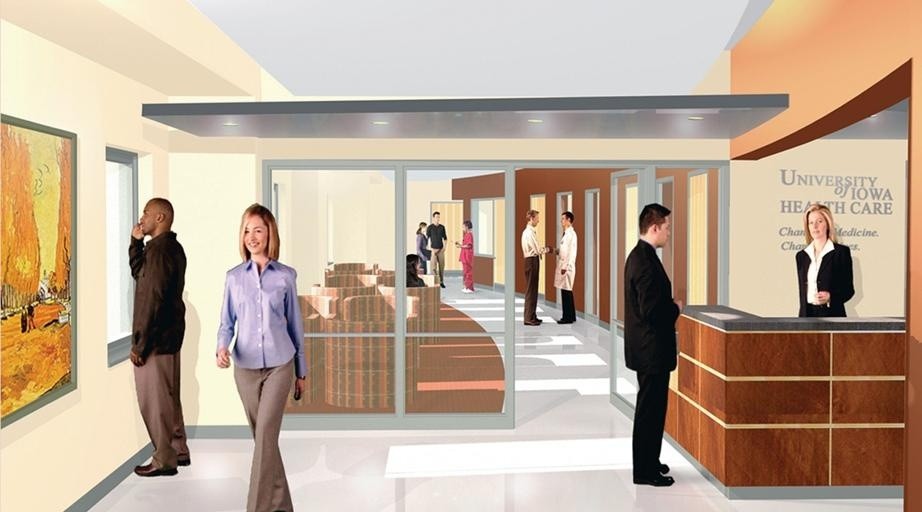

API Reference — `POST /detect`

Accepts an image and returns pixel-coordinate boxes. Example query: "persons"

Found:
[128,198,190,476]
[426,211,447,288]
[521,210,549,326]
[623,203,684,486]
[216,203,308,512]
[795,204,855,317]
[21,303,34,333]
[550,212,578,324]
[406,254,428,286]
[455,220,476,292]
[415,222,428,275]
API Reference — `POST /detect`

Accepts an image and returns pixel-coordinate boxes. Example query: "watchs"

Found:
[297,376,306,380]
